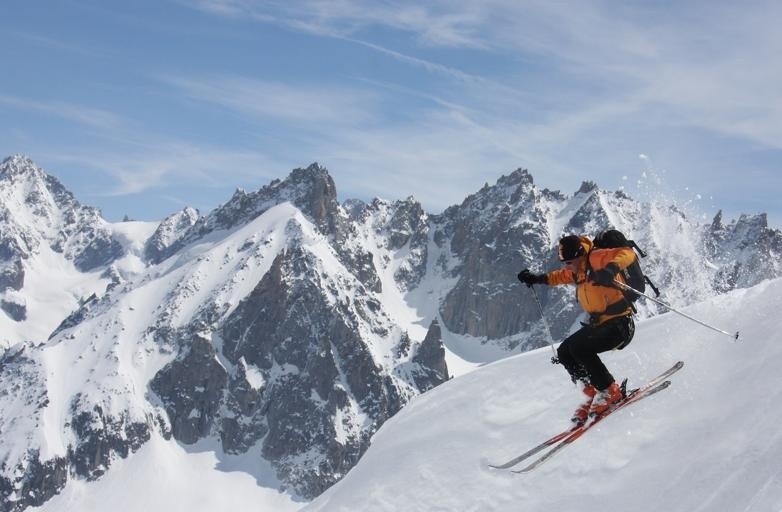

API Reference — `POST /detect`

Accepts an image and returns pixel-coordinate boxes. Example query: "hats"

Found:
[558,235,587,262]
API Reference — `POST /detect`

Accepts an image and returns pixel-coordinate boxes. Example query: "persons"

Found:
[518,229,640,424]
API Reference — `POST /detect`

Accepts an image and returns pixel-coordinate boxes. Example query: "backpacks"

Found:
[571,229,645,302]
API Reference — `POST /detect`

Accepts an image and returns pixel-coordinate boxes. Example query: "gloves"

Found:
[517,268,549,287]
[589,262,620,287]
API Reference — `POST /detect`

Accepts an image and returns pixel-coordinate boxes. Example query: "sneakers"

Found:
[571,384,598,423]
[588,382,624,418]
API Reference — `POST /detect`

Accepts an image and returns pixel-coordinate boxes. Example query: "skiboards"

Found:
[487,361,684,473]
